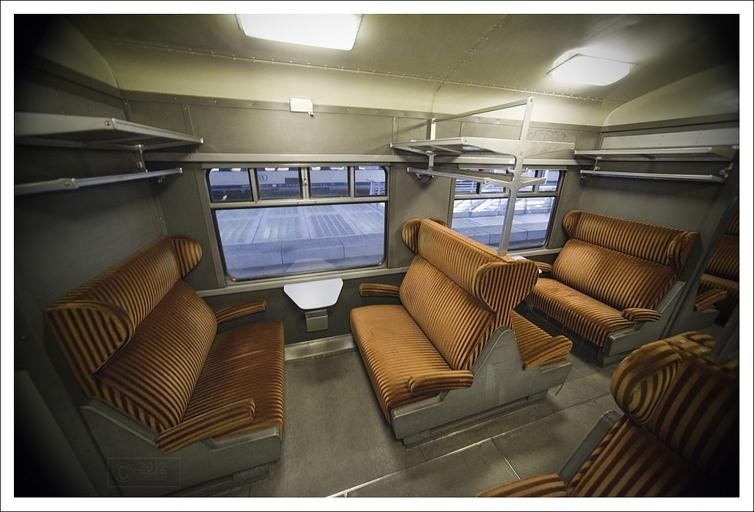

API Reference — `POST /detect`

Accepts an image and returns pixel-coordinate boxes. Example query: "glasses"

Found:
[229,13,363,52]
[546,51,638,87]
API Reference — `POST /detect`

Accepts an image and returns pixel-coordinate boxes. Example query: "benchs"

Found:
[348,207,739,447]
[39,233,286,497]
[475,331,739,498]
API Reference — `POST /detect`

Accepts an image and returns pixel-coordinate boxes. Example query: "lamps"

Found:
[546,51,638,87]
[229,13,363,52]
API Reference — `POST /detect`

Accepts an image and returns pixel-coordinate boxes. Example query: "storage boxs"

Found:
[39,233,286,497]
